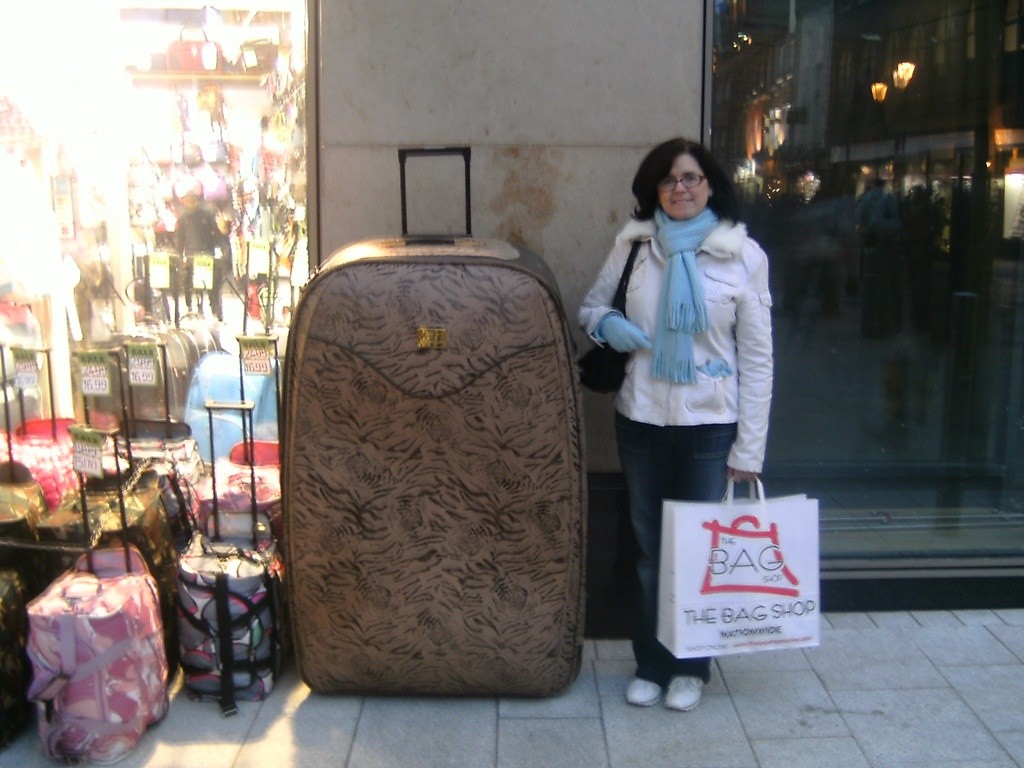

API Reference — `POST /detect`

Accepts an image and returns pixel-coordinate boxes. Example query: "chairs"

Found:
[243,222,301,334]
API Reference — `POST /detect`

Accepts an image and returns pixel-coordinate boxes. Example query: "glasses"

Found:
[657,174,707,190]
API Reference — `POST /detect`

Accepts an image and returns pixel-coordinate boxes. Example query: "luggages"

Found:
[0,309,281,768]
[280,145,589,699]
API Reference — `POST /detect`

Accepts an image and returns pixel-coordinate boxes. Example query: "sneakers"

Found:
[626,678,662,707]
[664,676,705,712]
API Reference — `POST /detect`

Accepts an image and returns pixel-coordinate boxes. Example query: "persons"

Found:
[170,192,231,327]
[725,164,945,341]
[576,136,774,710]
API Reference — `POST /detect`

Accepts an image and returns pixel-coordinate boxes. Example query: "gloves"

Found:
[699,358,732,380]
[599,314,652,352]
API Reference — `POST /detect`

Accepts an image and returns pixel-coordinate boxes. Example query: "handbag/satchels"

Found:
[115,17,279,236]
[656,475,820,659]
[576,240,643,394]
